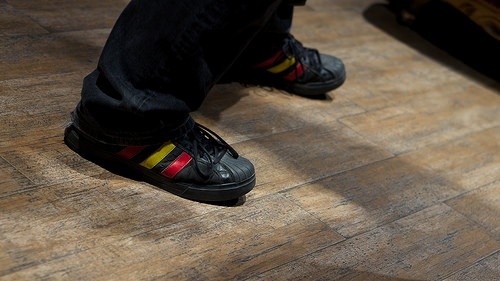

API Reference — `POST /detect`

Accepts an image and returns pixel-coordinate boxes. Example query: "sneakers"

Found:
[65,98,256,199]
[245,33,346,92]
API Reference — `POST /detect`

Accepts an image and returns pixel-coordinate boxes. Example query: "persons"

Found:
[64,0,347,204]
